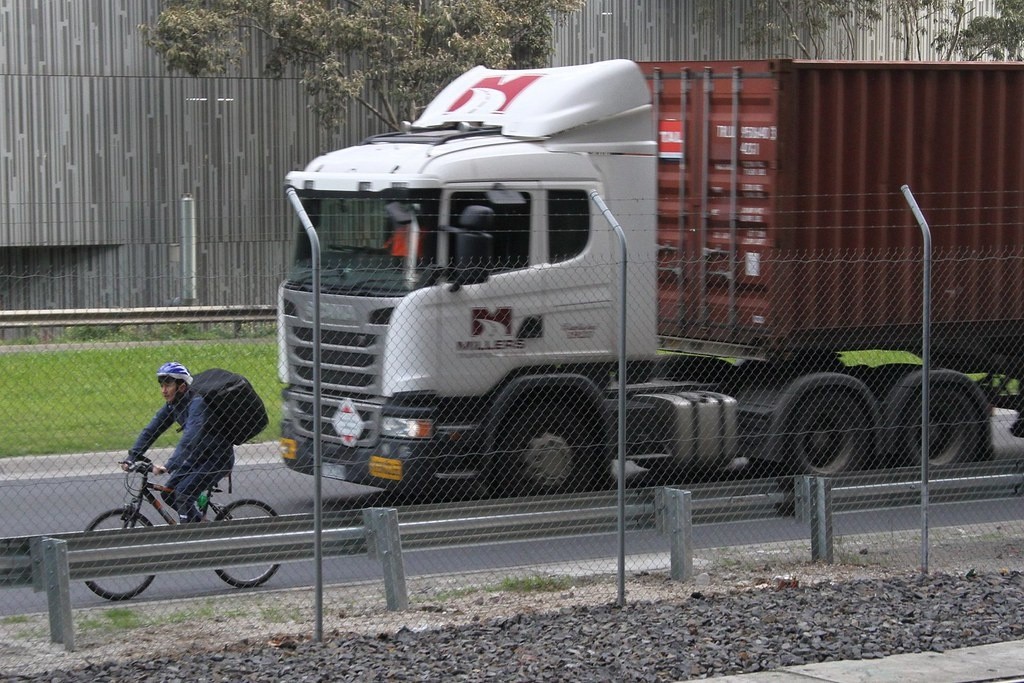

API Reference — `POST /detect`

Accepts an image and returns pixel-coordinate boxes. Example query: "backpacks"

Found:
[181,368,270,446]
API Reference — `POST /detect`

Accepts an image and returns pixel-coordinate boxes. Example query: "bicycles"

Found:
[79,455,288,600]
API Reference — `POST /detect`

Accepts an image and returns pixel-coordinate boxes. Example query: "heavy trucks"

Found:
[270,57,1024,503]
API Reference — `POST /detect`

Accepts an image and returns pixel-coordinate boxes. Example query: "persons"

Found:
[122,362,234,523]
[381,202,427,256]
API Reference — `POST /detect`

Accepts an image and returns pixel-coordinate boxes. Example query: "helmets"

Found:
[157,362,193,385]
